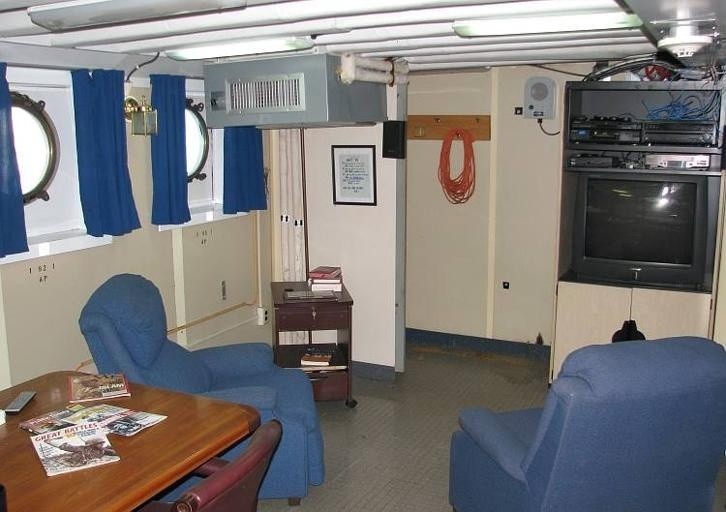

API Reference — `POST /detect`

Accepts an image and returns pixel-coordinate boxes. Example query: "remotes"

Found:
[5,392,36,414]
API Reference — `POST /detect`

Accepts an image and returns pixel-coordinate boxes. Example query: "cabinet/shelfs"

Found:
[271,282,356,407]
[548,282,715,385]
[562,81,726,175]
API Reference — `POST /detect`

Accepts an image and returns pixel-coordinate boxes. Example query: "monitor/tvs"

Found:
[572,173,708,284]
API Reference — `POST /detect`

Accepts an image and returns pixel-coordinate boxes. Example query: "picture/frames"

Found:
[331,145,377,206]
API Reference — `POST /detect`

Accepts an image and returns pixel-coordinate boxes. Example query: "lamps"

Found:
[165,38,311,61]
[452,15,643,38]
[27,0,246,31]
[124,95,161,139]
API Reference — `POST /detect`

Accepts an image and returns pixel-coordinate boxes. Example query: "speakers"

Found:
[383,121,405,158]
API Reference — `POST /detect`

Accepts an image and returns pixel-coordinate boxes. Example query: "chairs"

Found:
[450,337,726,512]
[78,274,324,505]
[133,419,284,512]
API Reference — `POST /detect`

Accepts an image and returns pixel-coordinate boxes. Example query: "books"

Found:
[300,350,333,365]
[18,372,168,477]
[308,266,343,292]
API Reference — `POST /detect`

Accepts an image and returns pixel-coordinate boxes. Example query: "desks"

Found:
[0,372,259,512]
